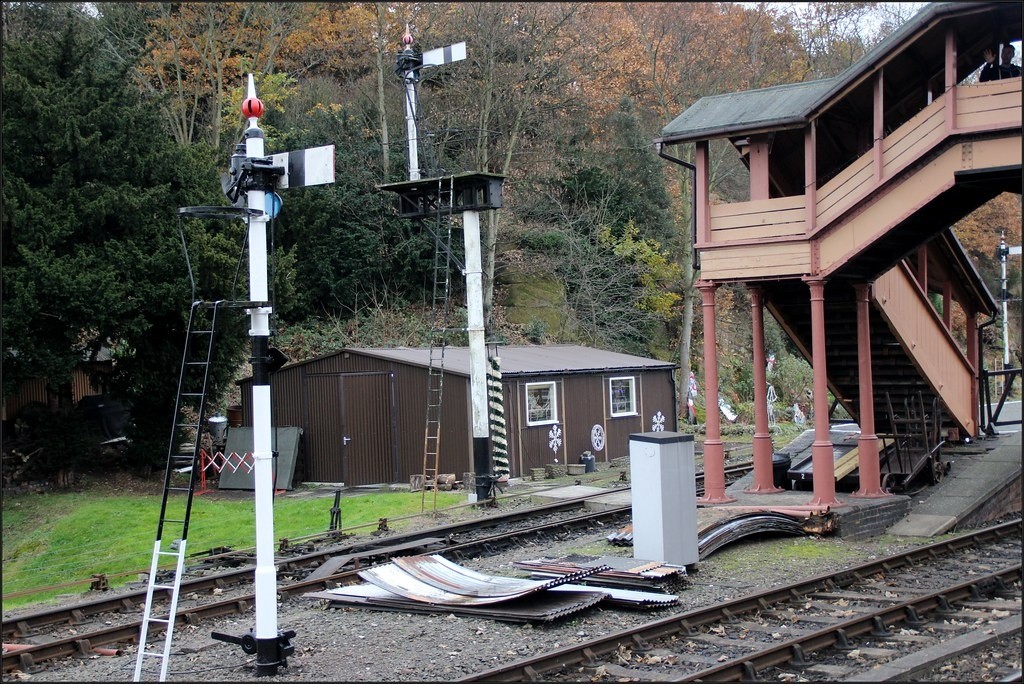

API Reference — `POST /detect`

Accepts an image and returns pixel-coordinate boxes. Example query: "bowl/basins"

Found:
[567,464,586,476]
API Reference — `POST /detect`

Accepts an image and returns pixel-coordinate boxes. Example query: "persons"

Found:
[979,44,1022,82]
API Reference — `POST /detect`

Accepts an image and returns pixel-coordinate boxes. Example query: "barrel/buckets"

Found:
[580,454,595,473]
